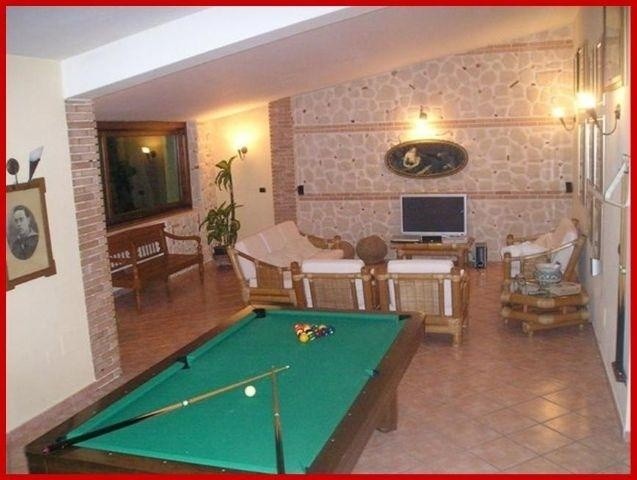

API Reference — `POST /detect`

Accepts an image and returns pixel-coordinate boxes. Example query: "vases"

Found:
[533,261,563,284]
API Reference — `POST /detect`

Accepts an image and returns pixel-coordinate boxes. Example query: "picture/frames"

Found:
[6,175,58,293]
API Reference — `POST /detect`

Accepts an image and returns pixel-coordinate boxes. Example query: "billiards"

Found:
[293,323,335,343]
[243,386,256,396]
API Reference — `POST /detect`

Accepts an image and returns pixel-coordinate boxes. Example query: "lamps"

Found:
[552,106,579,133]
[415,102,431,123]
[141,145,157,159]
[7,144,46,184]
[236,145,248,160]
[576,92,623,137]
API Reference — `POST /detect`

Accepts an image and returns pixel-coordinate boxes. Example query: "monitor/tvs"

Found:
[401,194,467,245]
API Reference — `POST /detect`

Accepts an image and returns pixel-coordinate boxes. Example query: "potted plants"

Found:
[198,149,247,269]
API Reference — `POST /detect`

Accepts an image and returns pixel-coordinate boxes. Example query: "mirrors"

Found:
[95,119,195,227]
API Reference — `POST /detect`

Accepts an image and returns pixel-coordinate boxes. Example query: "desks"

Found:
[388,236,477,259]
[24,300,428,478]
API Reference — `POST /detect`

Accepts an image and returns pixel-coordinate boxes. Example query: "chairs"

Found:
[290,259,380,314]
[374,257,471,349]
[498,216,591,291]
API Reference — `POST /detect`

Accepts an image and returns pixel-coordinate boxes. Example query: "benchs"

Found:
[105,222,205,312]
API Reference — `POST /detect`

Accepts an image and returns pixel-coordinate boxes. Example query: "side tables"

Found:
[500,281,593,338]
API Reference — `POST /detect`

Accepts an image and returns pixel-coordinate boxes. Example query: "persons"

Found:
[395,146,424,175]
[9,206,38,259]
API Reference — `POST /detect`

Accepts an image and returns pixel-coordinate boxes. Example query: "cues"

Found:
[42,366,288,474]
[616,173,630,371]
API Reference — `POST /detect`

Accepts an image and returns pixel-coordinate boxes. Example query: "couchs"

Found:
[225,218,356,308]
